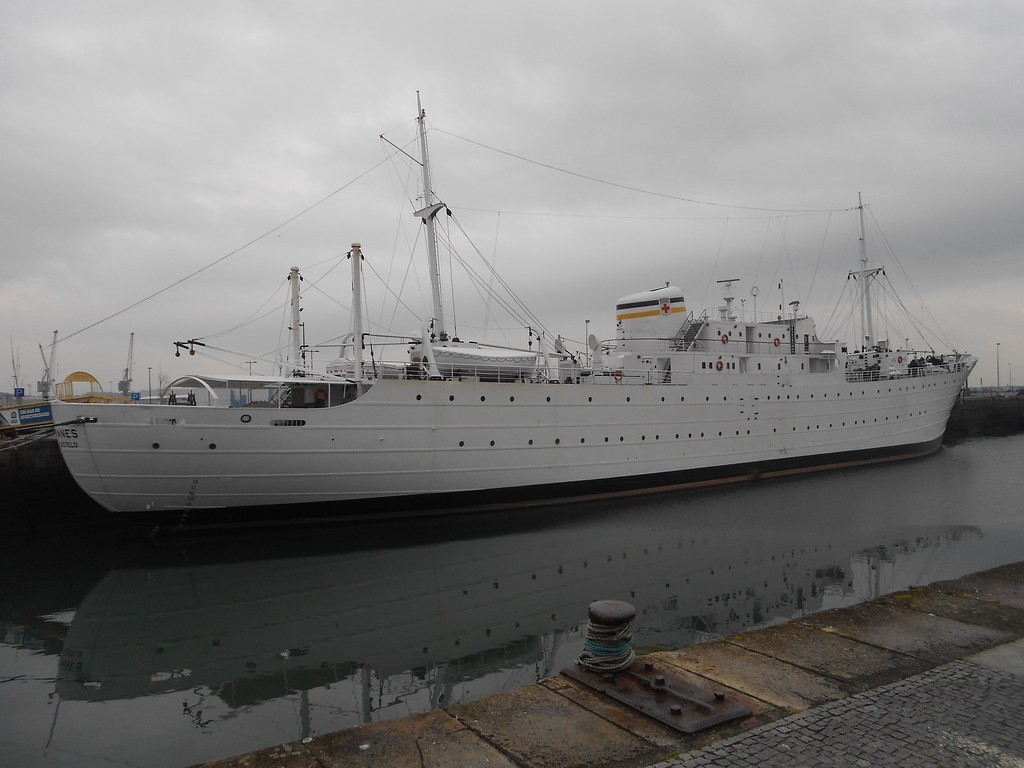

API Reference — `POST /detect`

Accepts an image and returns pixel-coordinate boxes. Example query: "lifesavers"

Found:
[315,389,328,402]
[774,338,780,347]
[898,356,902,363]
[722,334,728,344]
[716,361,723,371]
[614,370,622,381]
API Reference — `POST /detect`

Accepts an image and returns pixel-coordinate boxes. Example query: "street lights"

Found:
[996,342,1001,390]
[306,349,319,378]
[245,362,257,402]
[1008,363,1011,390]
[585,319,590,369]
[148,368,153,405]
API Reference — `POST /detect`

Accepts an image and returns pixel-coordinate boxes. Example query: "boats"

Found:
[35,88,980,549]
[44,446,988,755]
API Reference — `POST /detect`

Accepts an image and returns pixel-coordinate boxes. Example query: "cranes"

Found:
[118,332,134,396]
[36,330,58,401]
